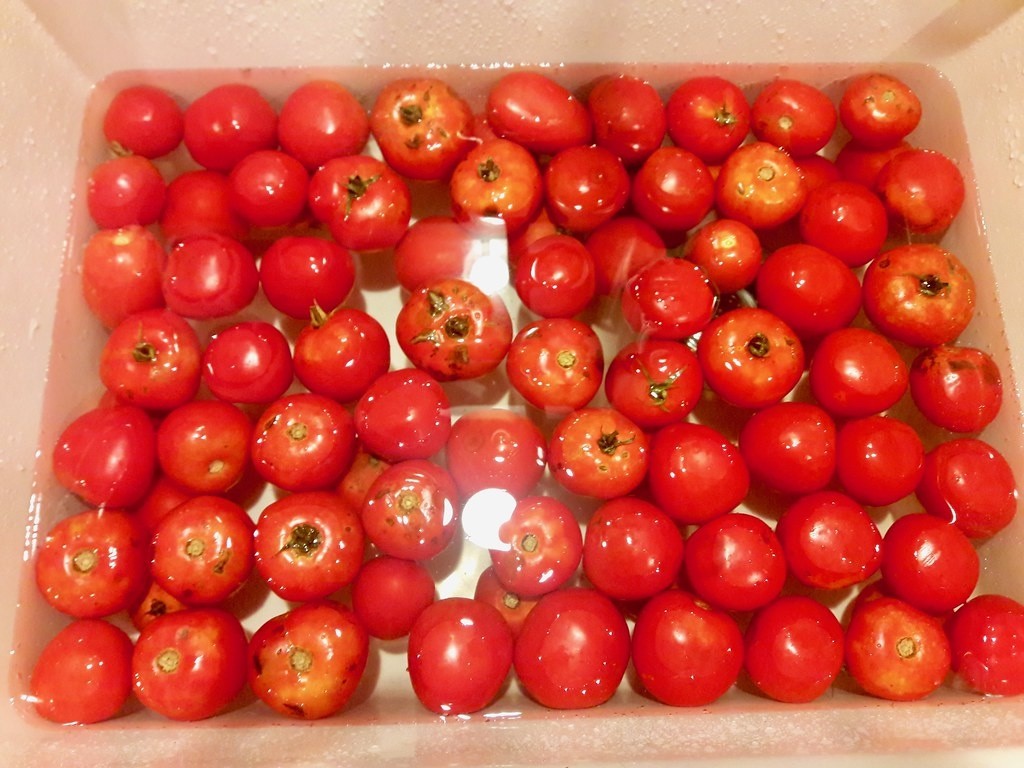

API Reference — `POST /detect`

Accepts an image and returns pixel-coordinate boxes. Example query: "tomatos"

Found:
[28,70,1024,723]
